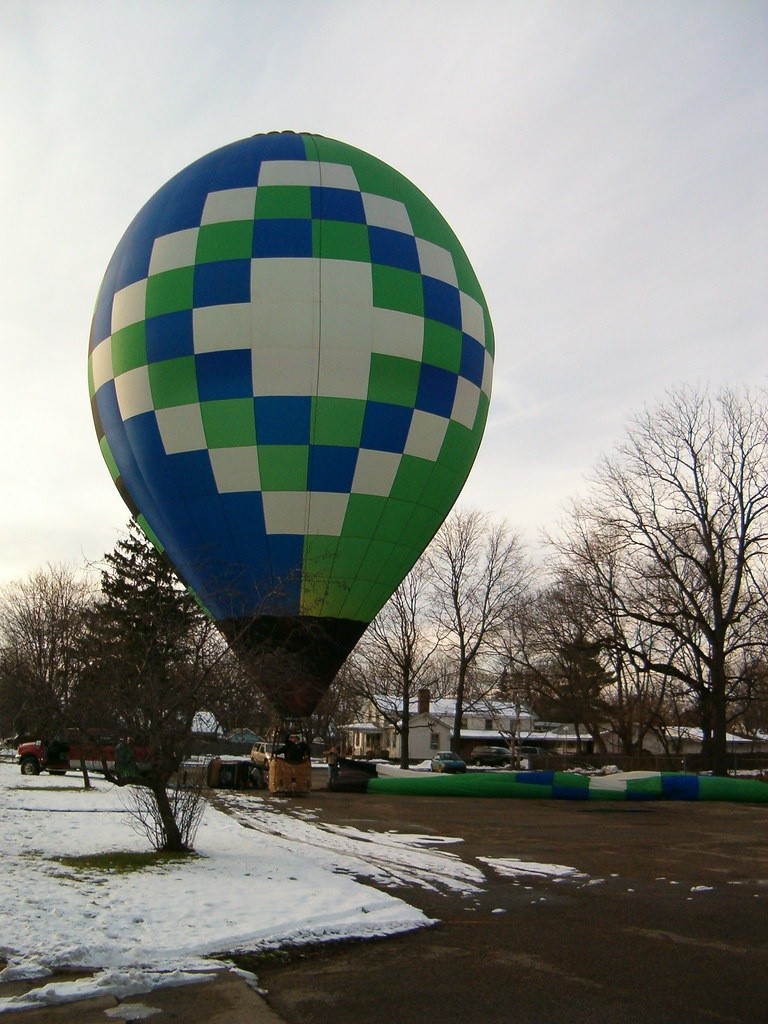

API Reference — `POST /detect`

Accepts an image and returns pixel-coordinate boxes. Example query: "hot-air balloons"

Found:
[87,131,494,793]
[310,753,768,807]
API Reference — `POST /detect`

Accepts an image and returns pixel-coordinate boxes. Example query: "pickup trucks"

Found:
[14,726,154,782]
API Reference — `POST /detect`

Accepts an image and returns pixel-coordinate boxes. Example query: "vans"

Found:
[251,741,286,768]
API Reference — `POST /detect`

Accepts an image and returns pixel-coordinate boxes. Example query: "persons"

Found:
[114,736,135,769]
[273,738,304,764]
[322,747,339,784]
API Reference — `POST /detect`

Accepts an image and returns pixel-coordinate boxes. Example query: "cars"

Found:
[429,751,468,773]
[469,745,514,767]
[514,747,553,757]
